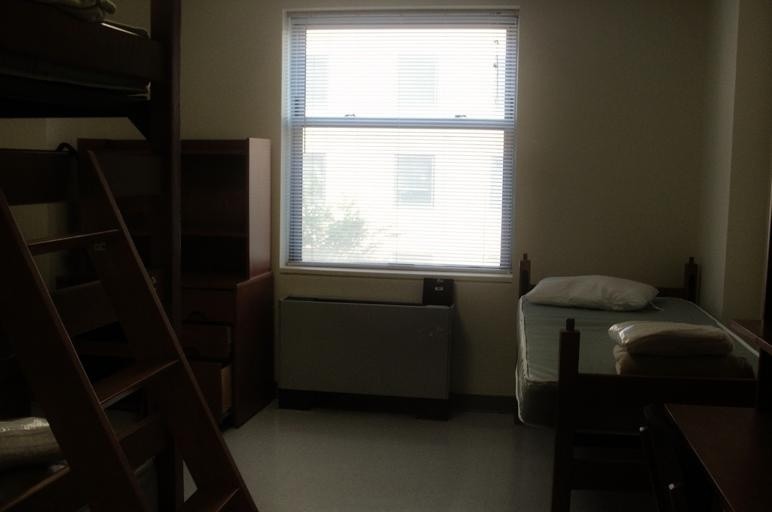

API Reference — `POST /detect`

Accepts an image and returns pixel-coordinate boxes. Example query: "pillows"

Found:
[609,314,735,356]
[527,270,661,313]
[610,345,756,381]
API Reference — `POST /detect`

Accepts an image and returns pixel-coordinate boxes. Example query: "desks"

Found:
[662,394,771,511]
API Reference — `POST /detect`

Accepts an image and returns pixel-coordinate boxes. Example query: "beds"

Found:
[0,0,264,509]
[507,253,770,512]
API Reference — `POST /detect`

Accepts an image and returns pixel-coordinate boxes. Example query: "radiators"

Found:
[273,296,458,424]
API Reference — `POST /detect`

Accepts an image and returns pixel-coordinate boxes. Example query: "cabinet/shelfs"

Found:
[55,270,277,429]
[72,134,273,280]
